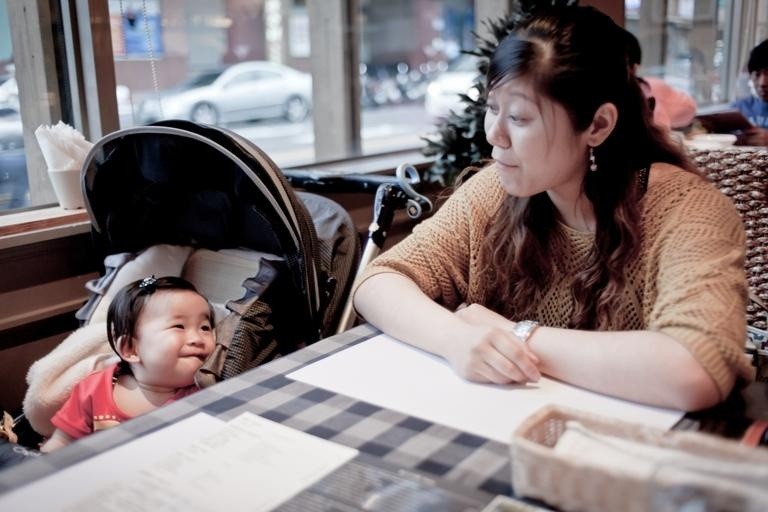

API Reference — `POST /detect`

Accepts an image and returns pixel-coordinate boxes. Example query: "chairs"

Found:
[689,149,768,380]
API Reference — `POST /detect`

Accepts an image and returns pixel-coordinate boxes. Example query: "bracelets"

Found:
[510,316,540,343]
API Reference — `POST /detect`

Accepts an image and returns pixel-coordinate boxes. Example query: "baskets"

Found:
[513,403,767,512]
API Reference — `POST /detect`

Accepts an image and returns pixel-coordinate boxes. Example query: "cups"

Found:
[48,170,83,211]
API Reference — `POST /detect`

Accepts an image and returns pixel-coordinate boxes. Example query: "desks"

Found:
[0,319,767,512]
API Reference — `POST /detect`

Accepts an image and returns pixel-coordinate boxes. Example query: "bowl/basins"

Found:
[691,134,737,146]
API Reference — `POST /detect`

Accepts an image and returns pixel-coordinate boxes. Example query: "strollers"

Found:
[0,121,435,470]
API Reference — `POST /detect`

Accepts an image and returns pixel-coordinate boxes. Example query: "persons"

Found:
[40,275,220,455]
[347,5,752,416]
[621,28,697,136]
[731,36,768,147]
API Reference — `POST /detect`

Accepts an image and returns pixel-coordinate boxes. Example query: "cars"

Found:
[139,61,312,125]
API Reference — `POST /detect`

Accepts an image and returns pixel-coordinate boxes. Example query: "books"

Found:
[693,108,757,142]
